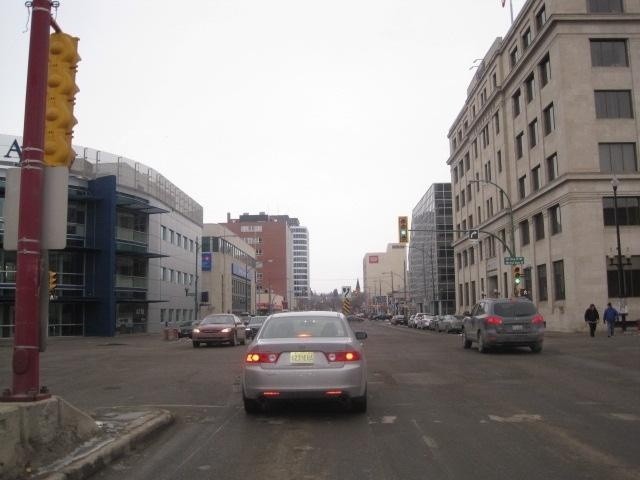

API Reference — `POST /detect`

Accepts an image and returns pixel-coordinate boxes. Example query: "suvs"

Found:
[461,298,545,354]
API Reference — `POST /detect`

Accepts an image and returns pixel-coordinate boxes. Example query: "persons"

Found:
[584,304,599,337]
[603,302,619,337]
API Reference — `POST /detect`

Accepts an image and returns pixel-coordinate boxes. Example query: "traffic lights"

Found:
[49,270,57,292]
[398,216,409,243]
[520,289,528,296]
[514,265,520,285]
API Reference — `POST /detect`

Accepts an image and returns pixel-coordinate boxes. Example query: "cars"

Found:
[232,311,269,341]
[347,312,465,334]
[178,320,201,338]
[115,315,134,334]
[192,313,246,348]
[242,310,368,415]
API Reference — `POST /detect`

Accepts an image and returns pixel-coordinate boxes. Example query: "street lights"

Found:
[504,257,525,265]
[195,234,241,320]
[409,245,436,315]
[469,179,519,297]
[607,173,632,332]
[246,259,274,312]
[382,272,406,316]
